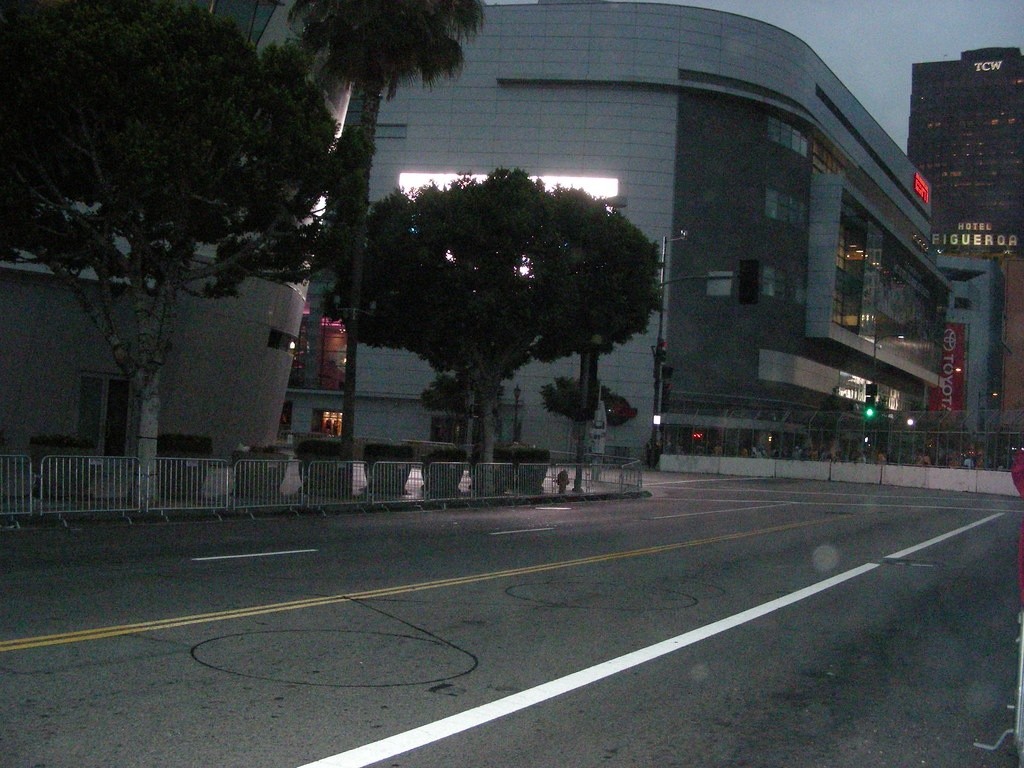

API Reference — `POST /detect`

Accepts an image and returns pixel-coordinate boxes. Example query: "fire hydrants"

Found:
[557,469,570,494]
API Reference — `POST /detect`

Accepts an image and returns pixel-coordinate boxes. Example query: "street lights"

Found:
[650,232,689,470]
[512,382,521,447]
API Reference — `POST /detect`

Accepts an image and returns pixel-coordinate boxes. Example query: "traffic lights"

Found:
[661,366,673,379]
[865,397,873,418]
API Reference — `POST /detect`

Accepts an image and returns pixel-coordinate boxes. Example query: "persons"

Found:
[557,468,570,494]
[676,443,983,470]
